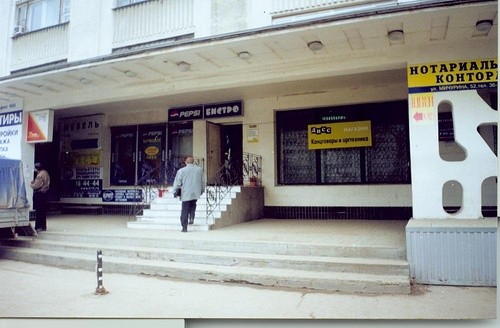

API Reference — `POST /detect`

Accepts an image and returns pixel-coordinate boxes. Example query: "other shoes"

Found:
[181,227,188,232]
[189,221,193,224]
[34,228,46,232]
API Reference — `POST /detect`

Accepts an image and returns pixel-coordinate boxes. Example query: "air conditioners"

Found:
[13,26,25,35]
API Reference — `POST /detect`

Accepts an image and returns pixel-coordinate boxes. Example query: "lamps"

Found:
[177,62,190,72]
[388,30,403,40]
[308,41,322,50]
[476,20,493,32]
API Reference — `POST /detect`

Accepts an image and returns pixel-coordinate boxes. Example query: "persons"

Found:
[29,162,50,232]
[172,157,205,233]
